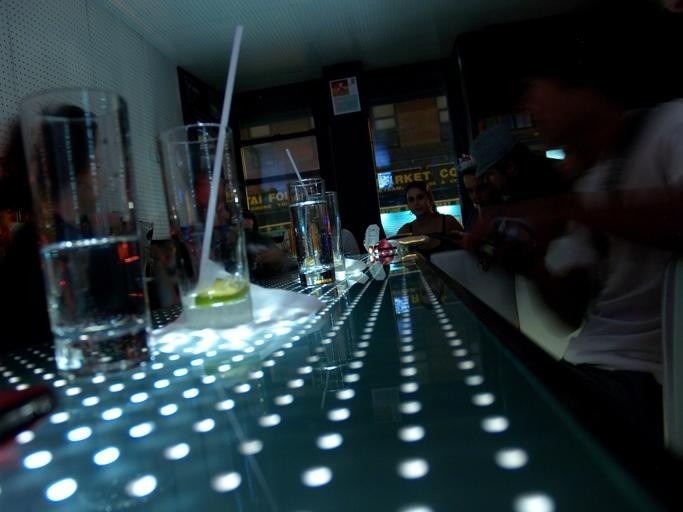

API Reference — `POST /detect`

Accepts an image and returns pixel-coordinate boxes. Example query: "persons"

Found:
[0,104,129,350]
[456,0,683,413]
[395,179,462,237]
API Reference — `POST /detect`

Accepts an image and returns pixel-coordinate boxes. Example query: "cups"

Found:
[23,89,154,379]
[160,122,252,329]
[287,178,346,287]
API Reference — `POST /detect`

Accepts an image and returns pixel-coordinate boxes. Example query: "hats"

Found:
[471,131,519,177]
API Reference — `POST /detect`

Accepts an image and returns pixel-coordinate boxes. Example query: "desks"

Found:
[0,252,658,511]
